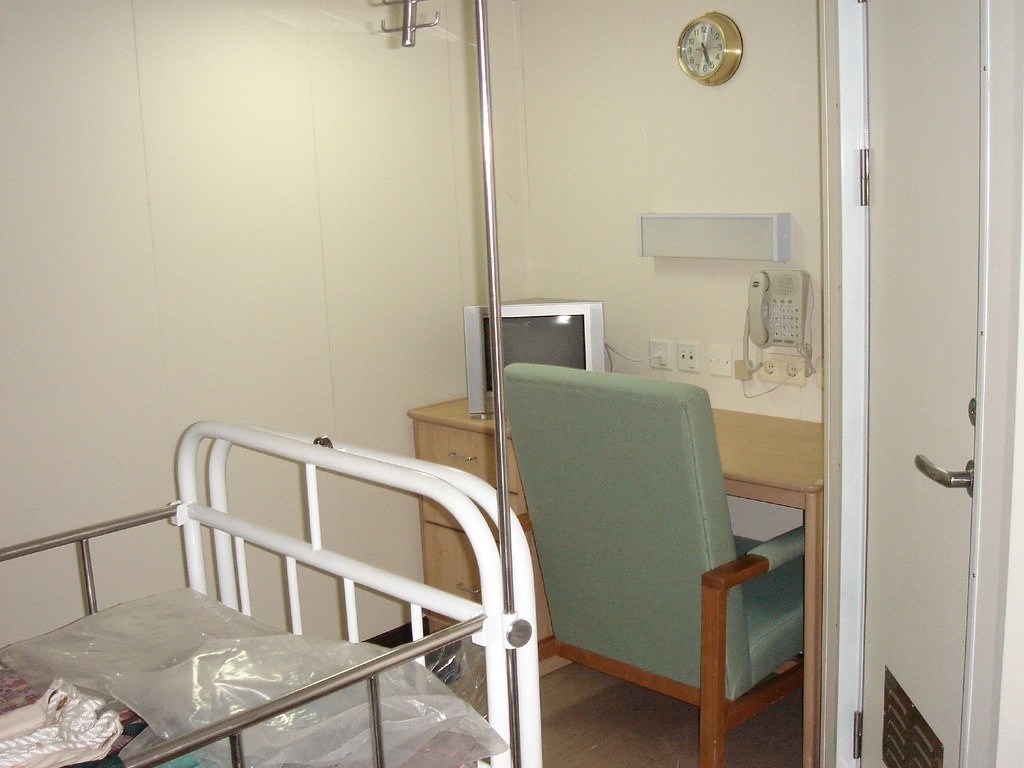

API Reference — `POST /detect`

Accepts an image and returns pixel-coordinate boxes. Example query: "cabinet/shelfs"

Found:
[406,397,823,768]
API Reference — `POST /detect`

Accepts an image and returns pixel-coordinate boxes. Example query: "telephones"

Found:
[744,266,813,350]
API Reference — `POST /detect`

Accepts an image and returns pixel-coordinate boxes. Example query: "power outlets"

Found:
[706,344,734,377]
[648,340,673,370]
[676,341,701,373]
[756,352,807,386]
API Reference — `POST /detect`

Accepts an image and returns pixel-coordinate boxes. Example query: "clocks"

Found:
[676,9,743,87]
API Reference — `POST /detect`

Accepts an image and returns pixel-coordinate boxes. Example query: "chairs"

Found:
[502,362,805,768]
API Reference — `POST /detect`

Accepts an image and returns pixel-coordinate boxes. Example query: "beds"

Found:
[0,418,544,768]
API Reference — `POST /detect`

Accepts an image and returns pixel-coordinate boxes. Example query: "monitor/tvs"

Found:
[463,297,605,420]
